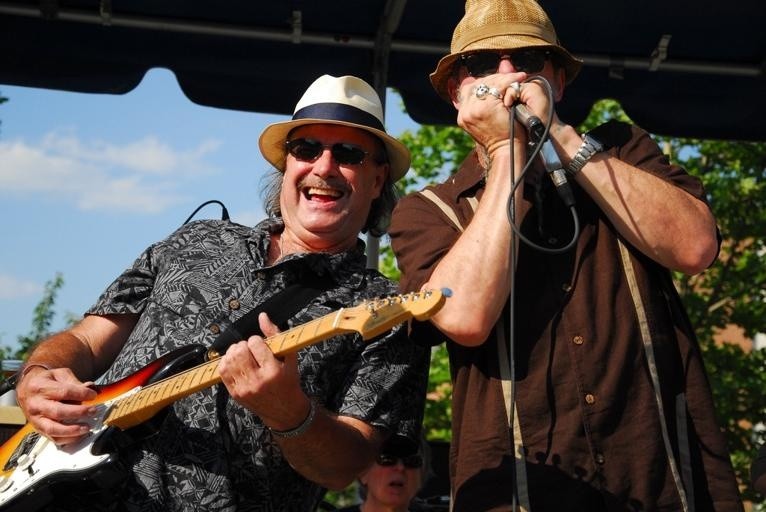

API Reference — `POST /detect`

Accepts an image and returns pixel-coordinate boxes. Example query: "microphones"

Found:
[508,101,546,143]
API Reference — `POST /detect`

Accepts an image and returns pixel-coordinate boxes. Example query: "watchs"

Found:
[566,133,603,176]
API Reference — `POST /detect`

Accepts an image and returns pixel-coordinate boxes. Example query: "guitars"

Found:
[0,288,452,512]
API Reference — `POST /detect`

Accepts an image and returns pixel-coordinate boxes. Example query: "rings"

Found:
[511,82,520,100]
[475,84,488,99]
[488,88,500,99]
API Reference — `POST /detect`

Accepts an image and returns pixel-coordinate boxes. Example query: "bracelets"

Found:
[269,399,315,440]
[22,363,51,378]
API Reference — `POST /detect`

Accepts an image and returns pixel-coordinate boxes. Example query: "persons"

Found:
[339,430,450,512]
[0,74,429,512]
[388,0,745,512]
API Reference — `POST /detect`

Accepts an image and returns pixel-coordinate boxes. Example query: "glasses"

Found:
[286,137,370,164]
[460,47,553,78]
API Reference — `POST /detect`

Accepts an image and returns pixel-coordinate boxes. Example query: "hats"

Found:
[427,0,587,105]
[258,73,412,185]
